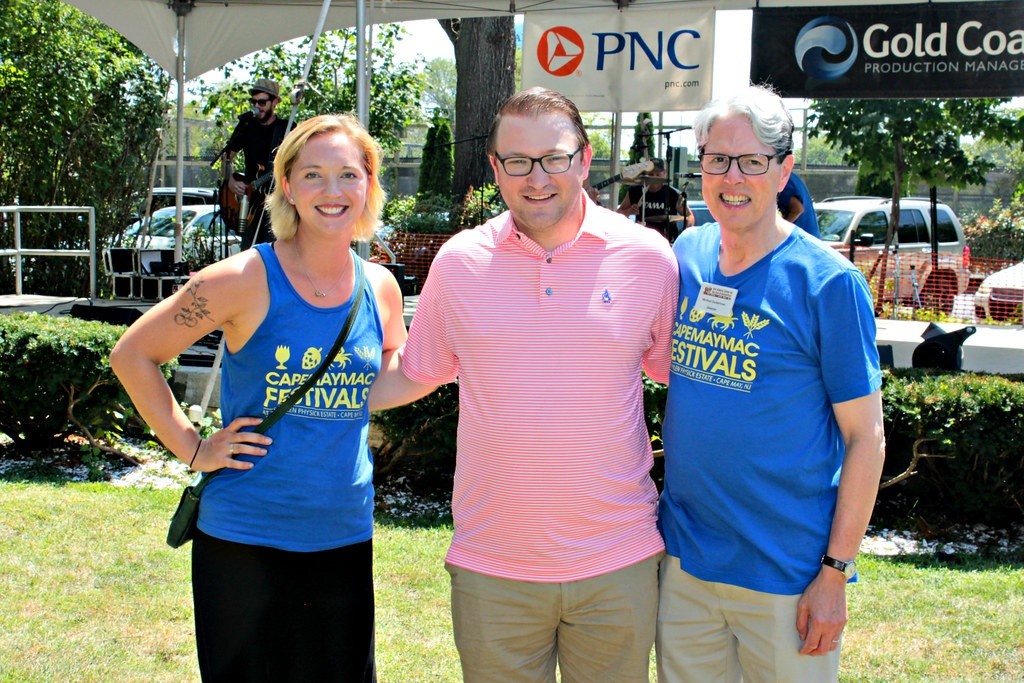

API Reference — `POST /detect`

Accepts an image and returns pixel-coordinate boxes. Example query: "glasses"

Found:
[698,149,789,175]
[249,98,270,106]
[494,146,583,177]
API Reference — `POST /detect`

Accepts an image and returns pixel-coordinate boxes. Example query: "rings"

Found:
[229,444,235,455]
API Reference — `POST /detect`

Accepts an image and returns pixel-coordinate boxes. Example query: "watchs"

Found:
[821,552,858,581]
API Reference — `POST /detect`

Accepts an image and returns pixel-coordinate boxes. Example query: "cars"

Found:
[113,206,241,260]
[126,187,218,209]
[973,257,1023,327]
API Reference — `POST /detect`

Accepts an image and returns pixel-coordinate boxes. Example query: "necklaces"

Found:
[293,233,353,297]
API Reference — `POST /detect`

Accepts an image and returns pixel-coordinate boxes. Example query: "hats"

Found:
[650,158,664,172]
[248,79,282,103]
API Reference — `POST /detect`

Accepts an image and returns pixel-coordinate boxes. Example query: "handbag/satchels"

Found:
[166,472,205,549]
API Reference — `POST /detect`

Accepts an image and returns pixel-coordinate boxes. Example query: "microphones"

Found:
[238,110,260,121]
[676,173,702,178]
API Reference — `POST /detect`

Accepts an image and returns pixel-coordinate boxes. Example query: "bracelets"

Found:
[189,439,203,468]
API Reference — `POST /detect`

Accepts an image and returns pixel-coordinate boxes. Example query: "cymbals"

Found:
[637,176,672,182]
[645,215,689,222]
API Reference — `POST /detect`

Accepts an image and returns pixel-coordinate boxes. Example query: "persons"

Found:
[107,110,412,683]
[655,86,885,683]
[617,154,694,252]
[776,172,820,240]
[221,76,301,250]
[364,86,683,683]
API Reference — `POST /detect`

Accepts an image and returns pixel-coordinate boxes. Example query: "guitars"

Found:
[219,168,274,229]
[584,156,654,193]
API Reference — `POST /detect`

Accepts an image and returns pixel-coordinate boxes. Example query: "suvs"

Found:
[814,194,970,317]
